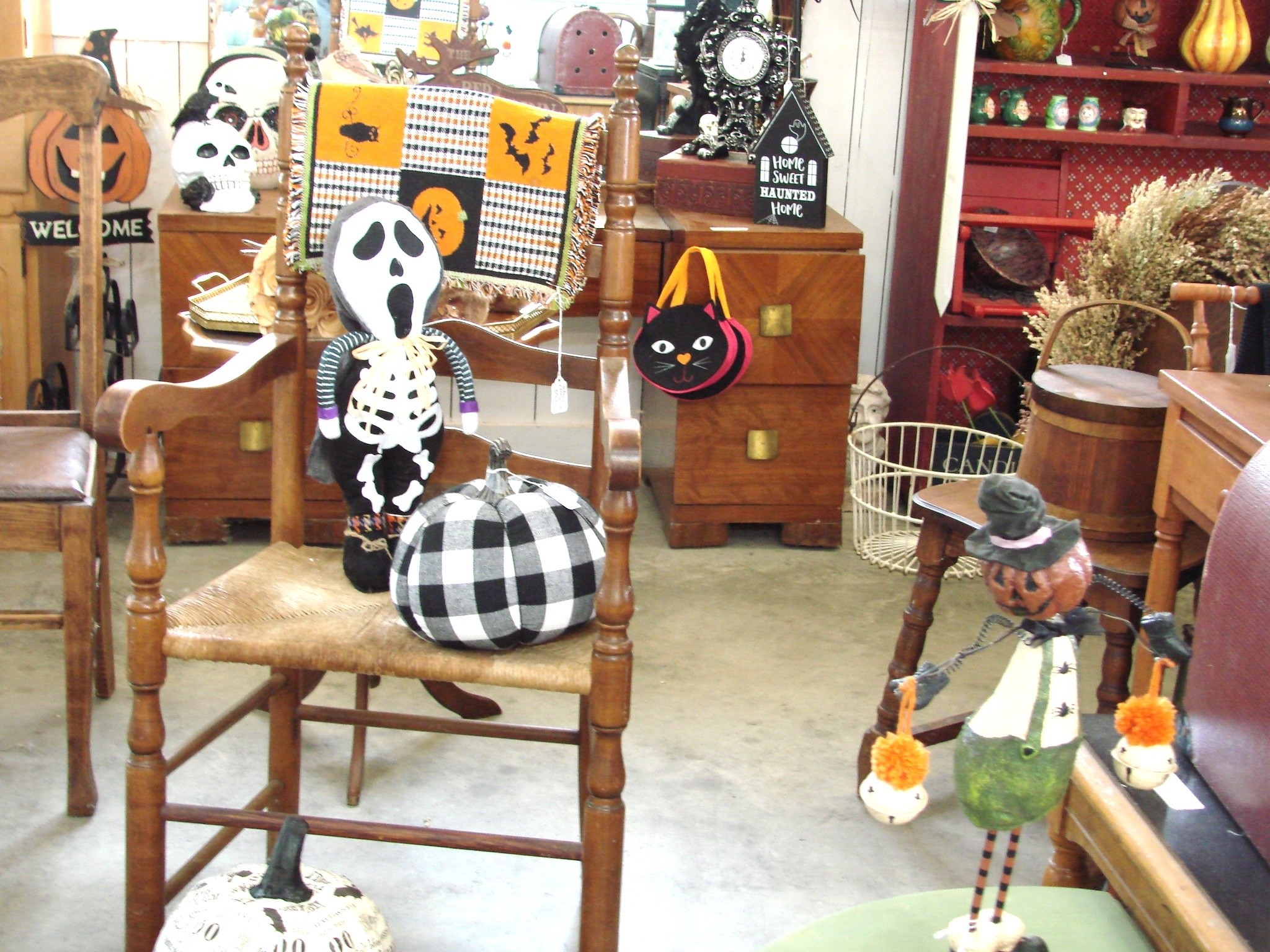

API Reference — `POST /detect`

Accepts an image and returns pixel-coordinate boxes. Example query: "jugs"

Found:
[1217,94,1266,139]
[985,0,1082,62]
[999,84,1032,127]
[969,84,994,125]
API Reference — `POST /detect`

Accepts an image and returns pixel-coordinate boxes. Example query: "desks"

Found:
[1044,366,1269,951]
[158,184,865,549]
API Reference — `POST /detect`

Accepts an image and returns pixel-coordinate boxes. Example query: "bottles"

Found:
[1045,94,1069,129]
[1076,96,1101,132]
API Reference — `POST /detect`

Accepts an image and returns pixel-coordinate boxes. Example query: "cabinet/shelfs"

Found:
[885,1,1269,516]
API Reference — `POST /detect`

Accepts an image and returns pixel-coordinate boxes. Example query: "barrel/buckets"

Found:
[1016,300,1193,544]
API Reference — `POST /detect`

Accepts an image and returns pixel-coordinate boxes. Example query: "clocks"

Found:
[684,1,799,164]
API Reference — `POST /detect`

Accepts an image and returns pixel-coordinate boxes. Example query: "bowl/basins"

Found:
[966,206,1050,292]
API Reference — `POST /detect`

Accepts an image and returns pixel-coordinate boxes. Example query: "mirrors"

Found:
[335,0,805,198]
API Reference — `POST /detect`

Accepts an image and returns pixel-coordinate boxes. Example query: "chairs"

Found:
[855,184,1265,796]
[92,23,639,952]
[1,56,119,823]
